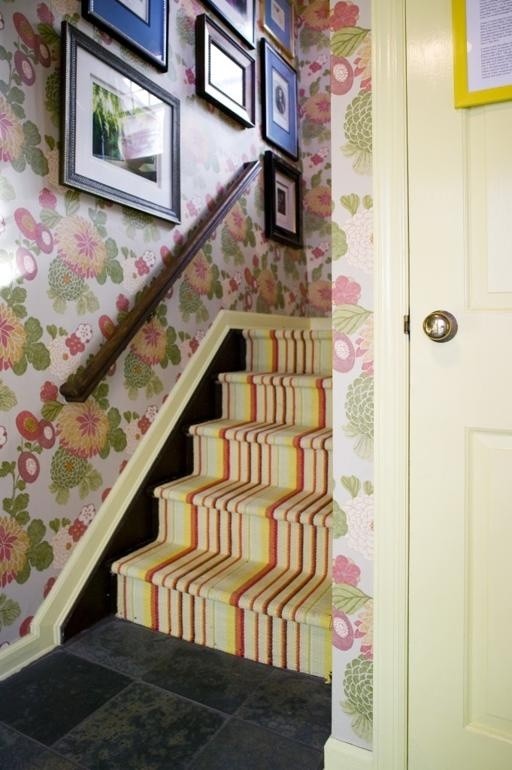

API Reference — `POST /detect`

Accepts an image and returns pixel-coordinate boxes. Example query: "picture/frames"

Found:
[200,0,257,50]
[261,0,295,59]
[260,37,299,162]
[264,150,303,249]
[196,13,256,128]
[82,0,169,73]
[451,0,512,109]
[59,19,182,225]
